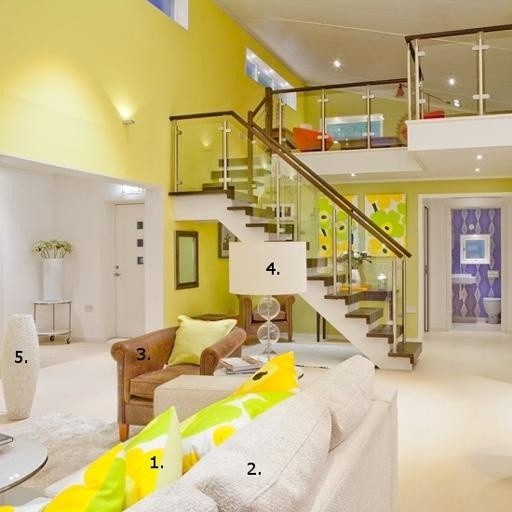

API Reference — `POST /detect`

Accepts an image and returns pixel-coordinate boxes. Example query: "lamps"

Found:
[394,82,451,114]
[123,119,136,125]
[228,239,309,374]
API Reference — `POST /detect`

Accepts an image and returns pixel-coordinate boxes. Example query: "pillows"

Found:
[180,392,297,478]
[167,313,237,368]
[125,407,187,507]
[226,352,300,394]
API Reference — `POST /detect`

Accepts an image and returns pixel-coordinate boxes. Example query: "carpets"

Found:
[0,407,145,509]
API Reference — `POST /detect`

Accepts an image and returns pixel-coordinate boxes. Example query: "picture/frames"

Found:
[460,234,490,266]
[174,229,199,291]
[218,222,237,258]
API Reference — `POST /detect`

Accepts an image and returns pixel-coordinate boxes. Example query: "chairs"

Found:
[237,293,294,340]
[112,312,248,443]
[402,109,446,141]
[292,127,333,151]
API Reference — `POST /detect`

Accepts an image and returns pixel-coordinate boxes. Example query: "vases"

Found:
[42,263,64,303]
[351,269,360,287]
[0,311,40,422]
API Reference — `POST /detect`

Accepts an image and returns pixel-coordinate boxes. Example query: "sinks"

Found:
[451,276,480,285]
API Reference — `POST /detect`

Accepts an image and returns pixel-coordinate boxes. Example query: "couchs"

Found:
[0,353,410,512]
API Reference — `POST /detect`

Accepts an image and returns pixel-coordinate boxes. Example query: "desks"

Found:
[336,136,407,149]
[316,289,399,342]
[264,128,295,152]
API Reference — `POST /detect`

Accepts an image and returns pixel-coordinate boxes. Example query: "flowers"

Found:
[33,239,73,264]
[337,245,372,269]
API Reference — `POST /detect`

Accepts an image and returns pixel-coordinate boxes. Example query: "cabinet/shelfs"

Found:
[33,300,72,345]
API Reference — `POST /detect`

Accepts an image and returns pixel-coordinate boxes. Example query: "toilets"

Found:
[483,297,501,324]
